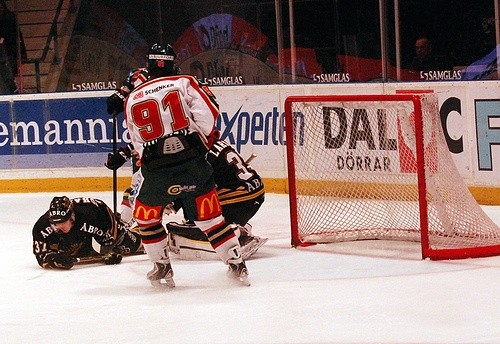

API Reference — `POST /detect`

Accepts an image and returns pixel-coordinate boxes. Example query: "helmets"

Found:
[49,196,74,224]
[148,41,176,70]
[123,67,151,93]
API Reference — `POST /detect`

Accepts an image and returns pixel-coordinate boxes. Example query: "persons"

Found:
[124,43,251,288]
[32,196,144,270]
[409,38,442,81]
[105,67,196,225]
[167,124,267,260]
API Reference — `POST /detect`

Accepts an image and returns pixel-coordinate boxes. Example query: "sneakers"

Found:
[238,227,269,259]
[147,246,175,288]
[227,245,250,286]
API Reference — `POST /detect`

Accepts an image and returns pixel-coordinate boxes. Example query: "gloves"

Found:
[105,254,122,264]
[43,252,73,270]
[107,88,128,117]
[105,147,130,170]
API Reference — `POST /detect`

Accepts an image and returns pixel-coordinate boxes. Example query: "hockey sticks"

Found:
[101,216,137,256]
[63,251,114,264]
[245,153,258,166]
[111,115,119,214]
